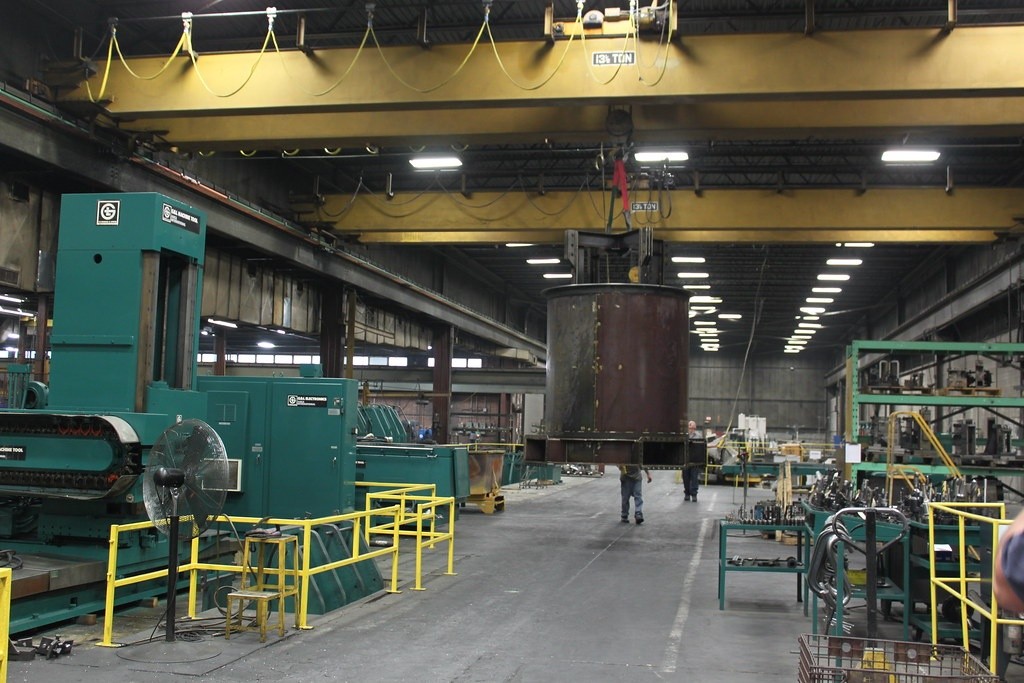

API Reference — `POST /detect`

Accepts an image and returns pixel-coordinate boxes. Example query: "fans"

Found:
[116,418,230,662]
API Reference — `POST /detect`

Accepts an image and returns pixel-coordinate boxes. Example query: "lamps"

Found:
[409,145,941,169]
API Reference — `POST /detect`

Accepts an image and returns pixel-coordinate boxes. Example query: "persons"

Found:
[994,508,1024,612]
[619,465,652,525]
[683,420,702,502]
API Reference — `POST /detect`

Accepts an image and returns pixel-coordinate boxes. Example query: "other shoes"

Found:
[685,495,690,501]
[621,518,629,523]
[636,517,644,524]
[692,496,697,502]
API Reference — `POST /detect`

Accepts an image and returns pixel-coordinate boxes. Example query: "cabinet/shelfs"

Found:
[802,500,909,683]
[718,518,809,617]
[909,519,983,653]
[846,340,1024,500]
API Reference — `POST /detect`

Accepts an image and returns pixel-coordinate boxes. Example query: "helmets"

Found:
[688,421,697,430]
